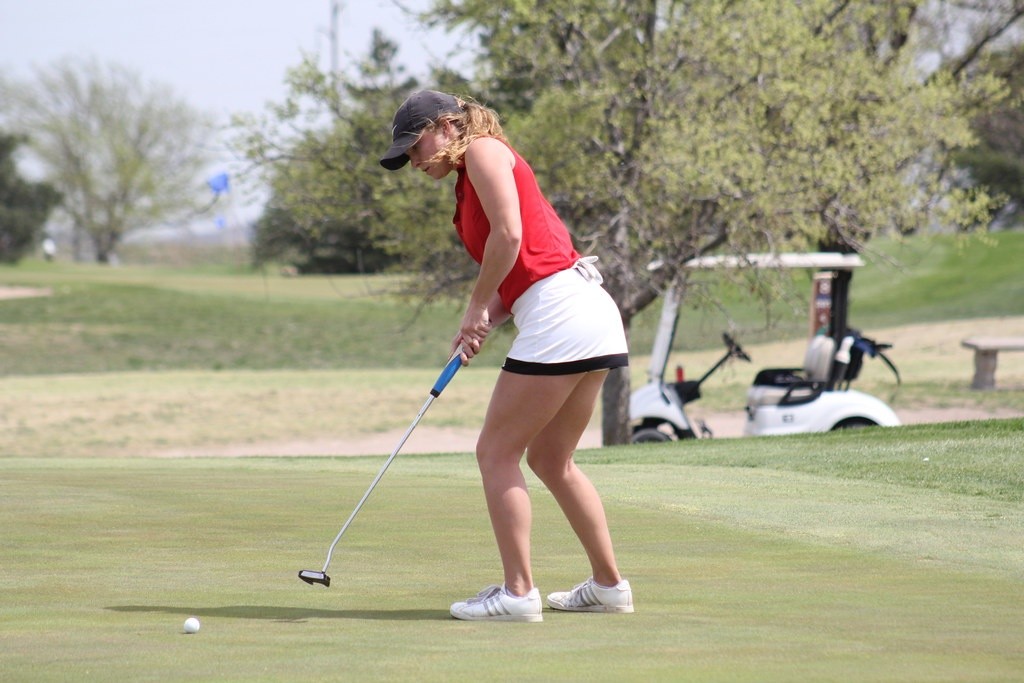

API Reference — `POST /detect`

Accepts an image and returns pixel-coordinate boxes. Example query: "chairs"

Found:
[747,334,833,406]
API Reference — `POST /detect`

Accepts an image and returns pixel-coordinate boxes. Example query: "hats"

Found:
[380,89,467,171]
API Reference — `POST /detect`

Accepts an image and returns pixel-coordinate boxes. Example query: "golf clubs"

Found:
[297,318,493,587]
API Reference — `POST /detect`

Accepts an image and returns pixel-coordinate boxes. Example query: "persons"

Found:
[379,90,634,624]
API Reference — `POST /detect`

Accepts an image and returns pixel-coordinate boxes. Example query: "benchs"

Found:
[959,335,1024,391]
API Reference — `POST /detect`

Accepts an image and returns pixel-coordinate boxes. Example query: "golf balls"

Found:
[183,617,201,634]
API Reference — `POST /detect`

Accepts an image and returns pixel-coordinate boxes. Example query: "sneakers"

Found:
[546,576,634,614]
[450,581,543,623]
